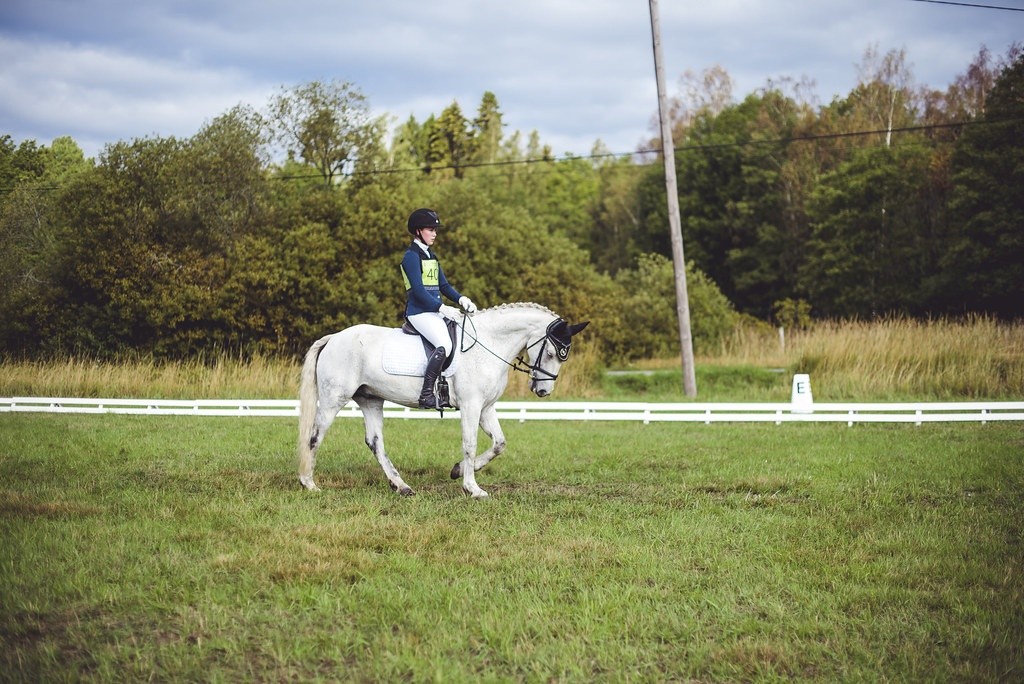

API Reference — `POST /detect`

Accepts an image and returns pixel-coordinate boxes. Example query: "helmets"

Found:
[408,208,443,234]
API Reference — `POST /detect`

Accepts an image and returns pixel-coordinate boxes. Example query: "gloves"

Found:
[458,296,477,314]
[438,303,463,323]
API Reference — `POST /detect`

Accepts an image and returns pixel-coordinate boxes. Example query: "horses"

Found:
[297,302,590,496]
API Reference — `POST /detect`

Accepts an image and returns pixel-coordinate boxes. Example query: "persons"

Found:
[399,208,478,413]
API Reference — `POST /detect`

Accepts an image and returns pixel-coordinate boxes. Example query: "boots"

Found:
[418,347,449,409]
